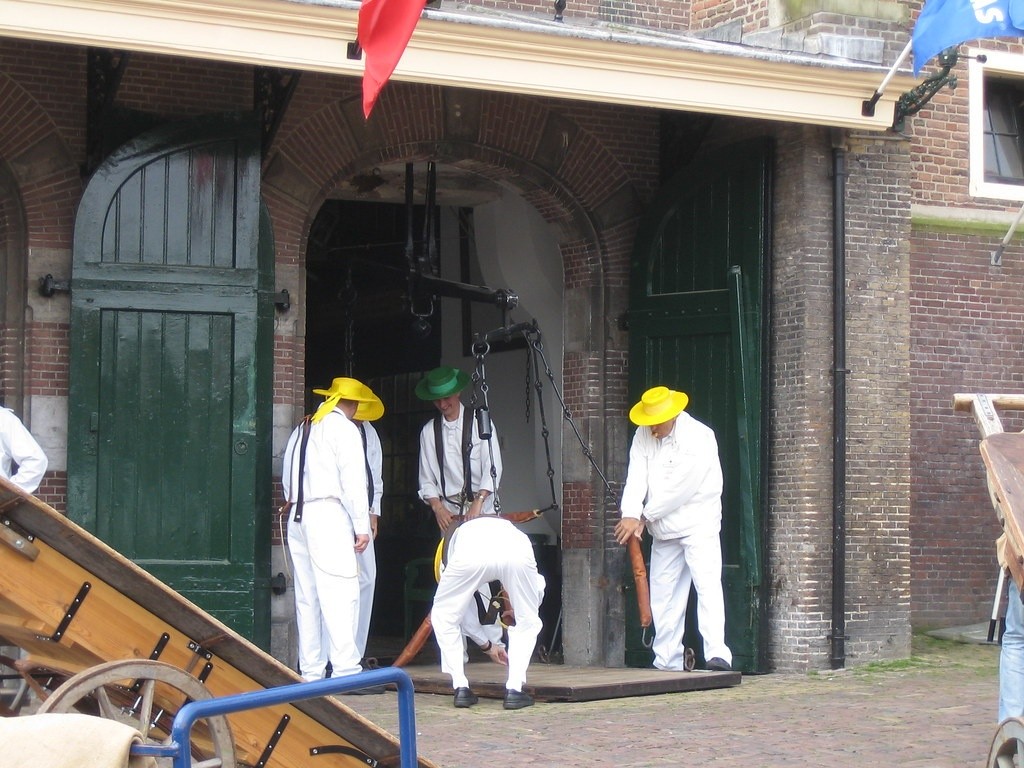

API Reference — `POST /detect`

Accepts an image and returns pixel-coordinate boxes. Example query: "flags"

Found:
[910,0,1024,79]
[358,0,427,119]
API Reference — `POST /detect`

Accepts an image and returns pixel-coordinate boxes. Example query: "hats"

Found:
[435,537,444,585]
[310,376,377,426]
[327,385,384,420]
[414,366,471,401]
[629,386,689,426]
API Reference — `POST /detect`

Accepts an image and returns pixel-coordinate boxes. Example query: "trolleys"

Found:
[954,393,1024,768]
[1,475,439,768]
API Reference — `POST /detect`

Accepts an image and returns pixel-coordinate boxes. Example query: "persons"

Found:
[0,404,48,495]
[325,394,383,669]
[614,386,733,671]
[281,376,386,695]
[430,512,547,708]
[414,367,503,663]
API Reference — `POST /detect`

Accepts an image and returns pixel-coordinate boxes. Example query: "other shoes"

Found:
[336,684,387,694]
[705,657,730,671]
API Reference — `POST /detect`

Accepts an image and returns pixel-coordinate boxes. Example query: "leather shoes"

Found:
[504,689,535,709]
[454,687,479,708]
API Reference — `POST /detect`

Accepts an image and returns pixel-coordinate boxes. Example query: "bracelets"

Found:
[479,640,492,654]
[473,492,485,502]
[642,515,648,523]
